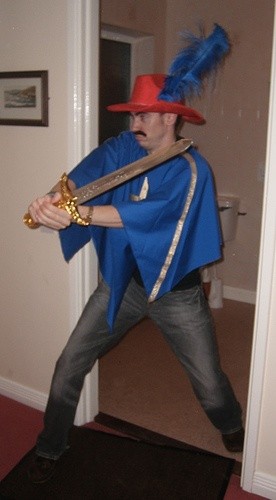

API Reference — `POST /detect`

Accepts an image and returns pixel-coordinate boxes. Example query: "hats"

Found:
[106,73,207,125]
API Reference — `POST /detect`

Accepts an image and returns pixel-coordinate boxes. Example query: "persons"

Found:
[28,75,245,484]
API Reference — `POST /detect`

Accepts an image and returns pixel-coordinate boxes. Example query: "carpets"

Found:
[0,425,236,500]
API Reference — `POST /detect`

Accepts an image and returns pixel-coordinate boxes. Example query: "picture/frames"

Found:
[0,69,49,127]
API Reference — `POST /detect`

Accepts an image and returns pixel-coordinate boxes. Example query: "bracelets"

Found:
[86,206,93,225]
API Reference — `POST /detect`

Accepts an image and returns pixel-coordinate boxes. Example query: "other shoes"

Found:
[25,446,72,484]
[223,427,245,452]
